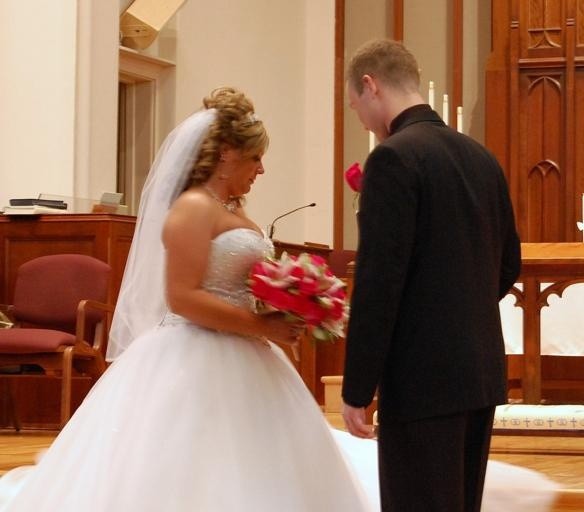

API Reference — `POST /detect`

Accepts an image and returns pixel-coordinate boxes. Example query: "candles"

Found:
[428,81,463,134]
[369,131,375,152]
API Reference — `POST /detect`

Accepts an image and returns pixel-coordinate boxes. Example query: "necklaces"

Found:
[204,185,247,213]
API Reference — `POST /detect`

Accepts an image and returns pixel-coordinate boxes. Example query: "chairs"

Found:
[0,254,119,431]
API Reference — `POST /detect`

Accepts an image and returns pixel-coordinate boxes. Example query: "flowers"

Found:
[246,251,351,344]
[344,161,363,193]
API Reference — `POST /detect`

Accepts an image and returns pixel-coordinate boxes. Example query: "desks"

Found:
[507,241,584,404]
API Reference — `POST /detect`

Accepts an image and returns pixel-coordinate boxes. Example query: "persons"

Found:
[0,86,558,511]
[339,36,518,509]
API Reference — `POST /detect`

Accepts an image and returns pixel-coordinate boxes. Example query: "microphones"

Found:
[269,202,316,239]
